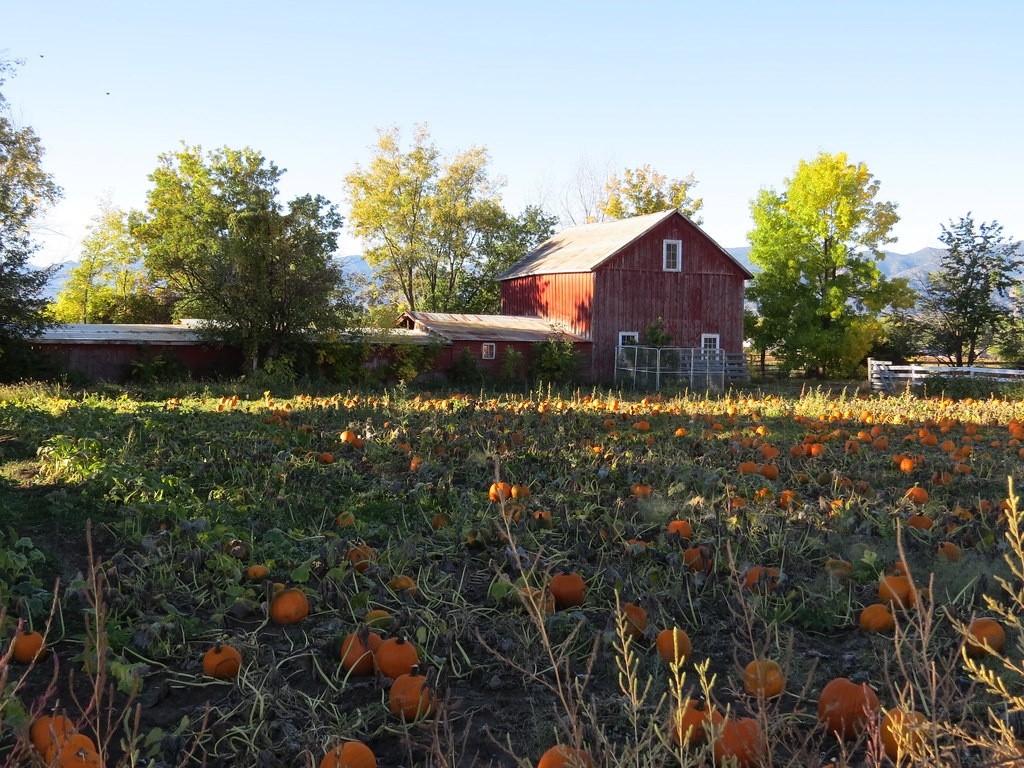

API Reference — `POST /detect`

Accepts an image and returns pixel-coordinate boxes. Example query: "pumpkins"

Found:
[13,392,1024,768]
